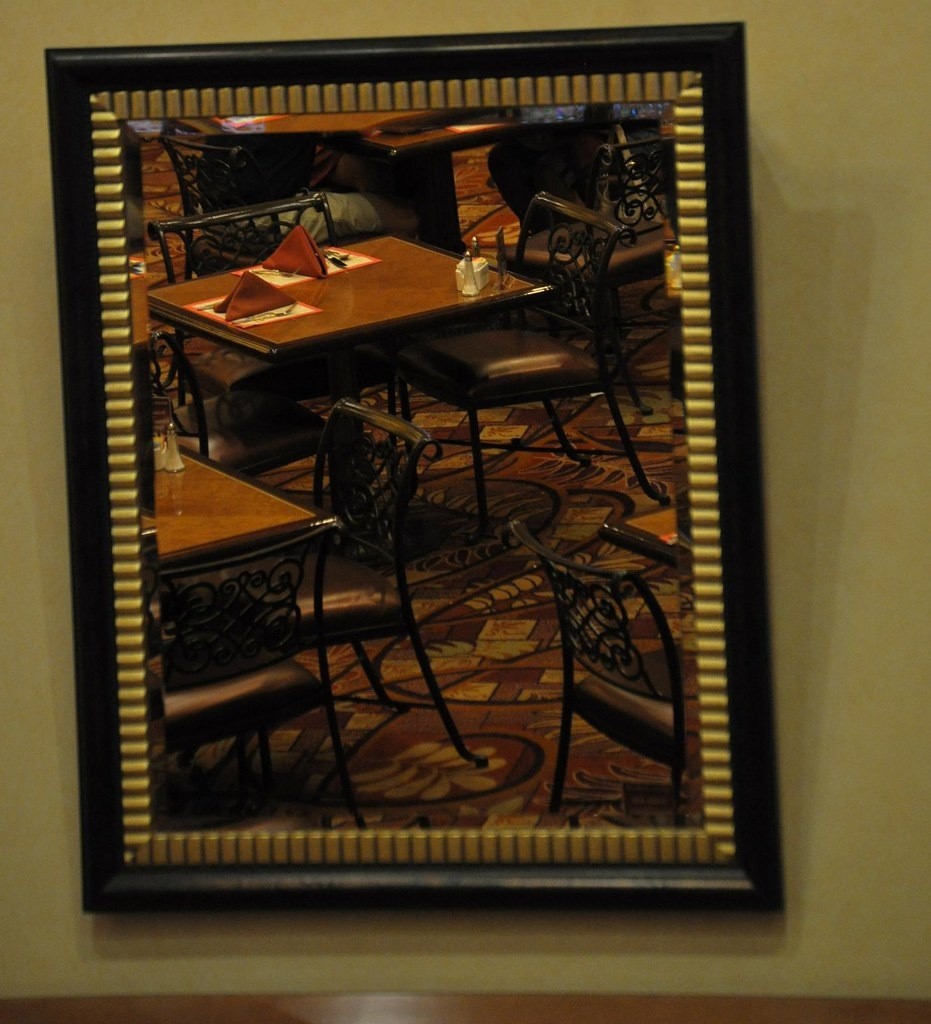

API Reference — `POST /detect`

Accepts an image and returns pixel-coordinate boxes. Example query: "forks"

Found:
[327,251,350,260]
[233,303,297,325]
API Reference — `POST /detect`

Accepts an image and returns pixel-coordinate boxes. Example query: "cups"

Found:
[153,442,166,471]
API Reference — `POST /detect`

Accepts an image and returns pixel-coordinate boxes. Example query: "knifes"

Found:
[322,251,347,267]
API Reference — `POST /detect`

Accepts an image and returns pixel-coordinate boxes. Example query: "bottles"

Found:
[471,235,481,258]
[462,251,480,297]
[164,422,186,473]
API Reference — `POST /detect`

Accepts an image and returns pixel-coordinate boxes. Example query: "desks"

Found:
[146,451,343,725]
[147,230,560,563]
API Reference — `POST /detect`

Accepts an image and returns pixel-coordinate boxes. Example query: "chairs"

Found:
[398,192,672,539]
[152,524,368,832]
[155,130,287,282]
[149,330,333,479]
[42,20,785,914]
[509,517,700,829]
[496,131,679,416]
[148,188,403,421]
[220,395,488,781]
